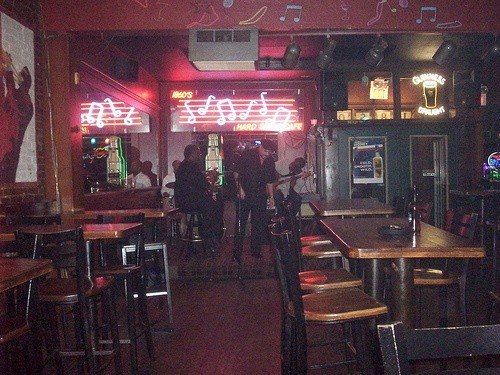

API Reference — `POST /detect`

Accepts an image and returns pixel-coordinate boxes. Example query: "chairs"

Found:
[0,213,156,375]
[266,189,500,375]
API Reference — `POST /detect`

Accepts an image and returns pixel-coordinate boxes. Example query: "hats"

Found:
[262,140,274,152]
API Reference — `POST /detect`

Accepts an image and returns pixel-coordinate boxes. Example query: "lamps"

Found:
[481,33,500,66]
[365,38,388,67]
[432,33,456,66]
[314,38,337,69]
[282,36,301,69]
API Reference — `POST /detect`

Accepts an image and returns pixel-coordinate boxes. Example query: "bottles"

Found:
[106,136,122,184]
[483,163,492,189]
[373,146,383,179]
[492,166,500,189]
[408,187,421,234]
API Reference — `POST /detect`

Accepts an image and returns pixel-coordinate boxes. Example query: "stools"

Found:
[179,209,214,262]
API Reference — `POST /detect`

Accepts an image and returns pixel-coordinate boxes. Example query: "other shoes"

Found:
[253,254,262,258]
[271,216,285,222]
[232,257,239,262]
[271,227,290,235]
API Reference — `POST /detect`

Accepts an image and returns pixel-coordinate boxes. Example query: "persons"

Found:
[174,144,219,238]
[233,139,276,260]
[128,160,158,188]
[161,159,188,239]
[276,157,306,214]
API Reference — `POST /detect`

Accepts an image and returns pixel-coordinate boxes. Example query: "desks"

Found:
[0,256,53,294]
[318,217,485,329]
[0,222,156,375]
[64,208,171,244]
[308,197,396,217]
